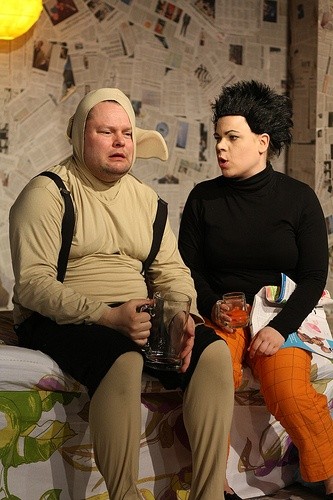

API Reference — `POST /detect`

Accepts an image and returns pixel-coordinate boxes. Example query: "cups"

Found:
[216,292,249,328]
[138,290,192,372]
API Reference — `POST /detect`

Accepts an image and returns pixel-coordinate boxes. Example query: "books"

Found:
[247,272,333,362]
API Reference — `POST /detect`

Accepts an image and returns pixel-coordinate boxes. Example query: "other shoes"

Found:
[223,486,242,500]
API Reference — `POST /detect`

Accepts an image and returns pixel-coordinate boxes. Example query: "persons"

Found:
[8,87,236,500]
[175,78,333,500]
[296,329,330,353]
